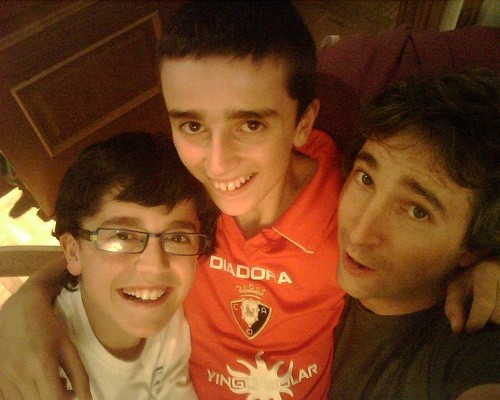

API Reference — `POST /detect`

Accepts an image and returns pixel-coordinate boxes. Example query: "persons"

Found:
[49,128,222,400]
[326,63,500,400]
[0,1,500,400]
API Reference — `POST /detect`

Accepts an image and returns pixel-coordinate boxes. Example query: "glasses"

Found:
[60,220,211,257]
